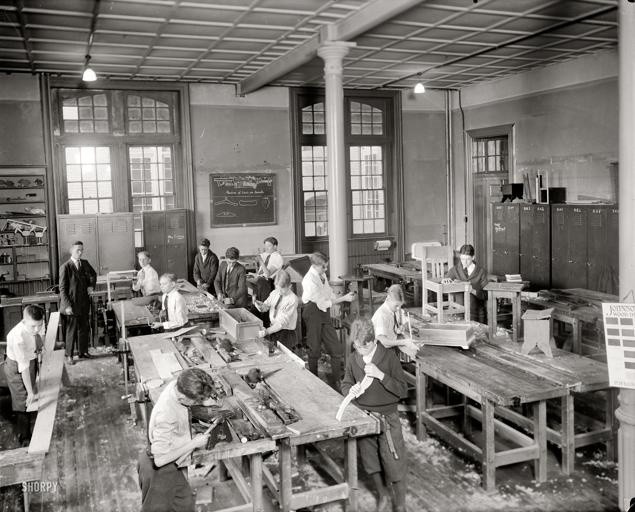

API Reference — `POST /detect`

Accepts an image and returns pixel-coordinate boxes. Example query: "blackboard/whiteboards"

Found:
[209,173,276,228]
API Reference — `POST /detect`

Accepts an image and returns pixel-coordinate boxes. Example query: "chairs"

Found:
[421,245,471,325]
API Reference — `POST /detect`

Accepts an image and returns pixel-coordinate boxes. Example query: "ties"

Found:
[36,334,42,352]
[258,254,271,275]
[319,274,325,284]
[464,267,468,279]
[394,313,399,335]
[225,268,230,291]
[274,295,282,318]
[164,294,168,321]
[188,407,193,465]
[78,260,80,269]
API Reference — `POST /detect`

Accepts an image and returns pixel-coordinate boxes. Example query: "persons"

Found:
[3,303,46,450]
[340,317,410,511]
[130,251,162,298]
[192,236,414,396]
[147,272,189,333]
[58,240,98,367]
[439,244,489,325]
[135,366,224,512]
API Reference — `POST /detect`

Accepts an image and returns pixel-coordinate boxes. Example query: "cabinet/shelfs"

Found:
[0,166,52,299]
[56,214,100,276]
[144,209,193,283]
[492,201,520,279]
[548,204,588,291]
[98,212,136,273]
[521,205,552,292]
[589,205,619,295]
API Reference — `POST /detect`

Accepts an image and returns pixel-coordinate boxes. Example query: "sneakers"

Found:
[377,495,406,512]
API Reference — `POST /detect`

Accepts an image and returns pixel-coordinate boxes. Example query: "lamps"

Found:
[414,72,425,94]
[83,53,98,81]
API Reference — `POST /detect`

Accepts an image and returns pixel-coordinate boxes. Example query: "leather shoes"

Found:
[79,352,97,359]
[66,356,75,365]
